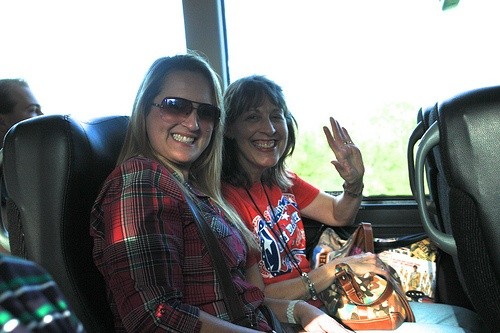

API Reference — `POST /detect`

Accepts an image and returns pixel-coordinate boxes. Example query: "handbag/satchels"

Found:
[319,222,416,330]
[258,304,308,333]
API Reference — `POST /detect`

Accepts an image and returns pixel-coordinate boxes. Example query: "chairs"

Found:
[407,86,500,333]
[0,113,130,333]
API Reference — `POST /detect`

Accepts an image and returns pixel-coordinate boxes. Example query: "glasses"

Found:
[150,96,221,126]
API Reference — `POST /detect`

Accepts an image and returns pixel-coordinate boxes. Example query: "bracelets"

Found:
[341,181,364,198]
[287,300,305,324]
[302,272,317,302]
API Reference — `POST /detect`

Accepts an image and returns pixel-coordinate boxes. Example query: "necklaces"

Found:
[236,175,303,276]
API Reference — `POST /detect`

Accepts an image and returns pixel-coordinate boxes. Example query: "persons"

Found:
[211,75,392,317]
[91,56,354,333]
[0,79,43,252]
[398,266,435,297]
[0,245,87,333]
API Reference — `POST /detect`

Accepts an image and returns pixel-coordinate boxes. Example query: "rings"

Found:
[379,265,386,271]
[345,142,351,145]
